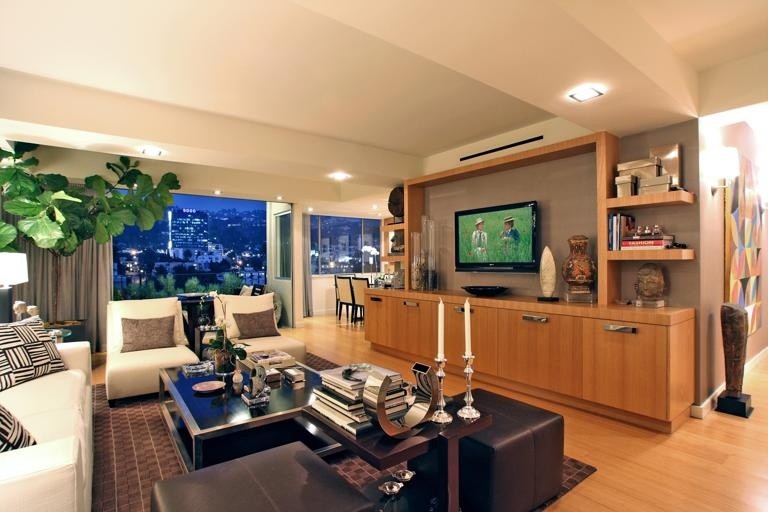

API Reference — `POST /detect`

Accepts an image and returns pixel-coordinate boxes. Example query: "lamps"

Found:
[0,251,28,290]
[707,142,741,198]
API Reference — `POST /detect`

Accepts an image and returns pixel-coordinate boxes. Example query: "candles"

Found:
[463,295,472,356]
[436,295,446,360]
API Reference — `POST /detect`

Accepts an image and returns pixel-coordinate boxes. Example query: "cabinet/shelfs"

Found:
[397,286,432,365]
[573,300,696,433]
[380,222,405,262]
[496,294,573,407]
[607,189,697,261]
[430,290,499,385]
[361,287,397,355]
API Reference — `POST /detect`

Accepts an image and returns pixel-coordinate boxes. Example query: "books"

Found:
[237,350,296,377]
[608,211,675,247]
[312,361,409,436]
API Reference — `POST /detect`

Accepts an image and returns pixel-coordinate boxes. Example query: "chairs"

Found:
[333,273,370,324]
[105,283,305,409]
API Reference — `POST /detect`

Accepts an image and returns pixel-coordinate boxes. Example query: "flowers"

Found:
[360,245,379,255]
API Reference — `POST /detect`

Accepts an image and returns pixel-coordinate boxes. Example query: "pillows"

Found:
[0,315,69,394]
[1,405,37,454]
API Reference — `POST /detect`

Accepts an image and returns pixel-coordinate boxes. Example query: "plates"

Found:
[461,286,509,297]
[192,380,227,393]
[388,187,404,217]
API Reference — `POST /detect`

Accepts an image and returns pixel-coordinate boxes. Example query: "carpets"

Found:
[91,350,598,512]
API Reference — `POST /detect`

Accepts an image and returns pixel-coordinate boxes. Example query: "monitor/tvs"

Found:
[454,200,541,275]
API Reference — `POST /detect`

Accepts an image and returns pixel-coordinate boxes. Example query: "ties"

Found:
[479,232,482,245]
[506,231,509,238]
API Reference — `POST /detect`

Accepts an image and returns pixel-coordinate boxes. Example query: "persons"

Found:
[472,218,488,257]
[26,305,39,317]
[12,301,27,322]
[498,216,520,243]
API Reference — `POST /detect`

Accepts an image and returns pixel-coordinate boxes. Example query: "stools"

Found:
[411,387,563,512]
[151,440,377,512]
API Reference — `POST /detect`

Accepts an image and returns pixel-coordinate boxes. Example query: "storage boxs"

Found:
[614,156,673,198]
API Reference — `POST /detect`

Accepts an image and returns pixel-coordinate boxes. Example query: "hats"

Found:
[504,216,515,223]
[475,218,484,226]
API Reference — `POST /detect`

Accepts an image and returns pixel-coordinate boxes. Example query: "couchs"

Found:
[0,314,94,510]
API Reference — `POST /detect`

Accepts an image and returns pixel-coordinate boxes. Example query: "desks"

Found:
[300,392,494,511]
[193,325,225,363]
